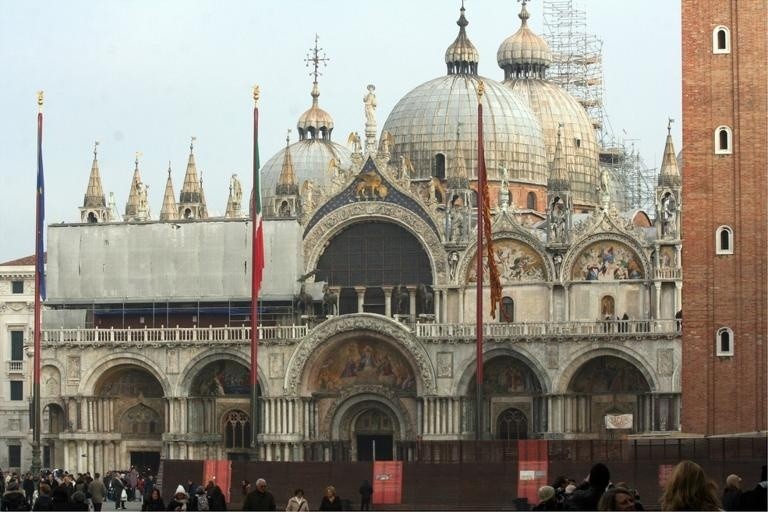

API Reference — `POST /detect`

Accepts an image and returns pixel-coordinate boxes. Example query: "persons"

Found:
[358,479,372,511]
[675,309,682,332]
[602,311,650,333]
[318,485,342,512]
[362,84,377,122]
[284,488,309,512]
[242,477,276,511]
[0,465,252,511]
[531,461,766,510]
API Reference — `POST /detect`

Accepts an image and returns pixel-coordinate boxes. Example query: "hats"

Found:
[538,486,555,502]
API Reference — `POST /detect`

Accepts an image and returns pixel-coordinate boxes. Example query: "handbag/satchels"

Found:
[120,489,127,502]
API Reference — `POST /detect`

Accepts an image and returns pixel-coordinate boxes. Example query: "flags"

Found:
[475,104,512,392]
[245,106,266,388]
[34,111,47,399]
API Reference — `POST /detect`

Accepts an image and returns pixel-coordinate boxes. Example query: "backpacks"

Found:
[195,491,209,511]
